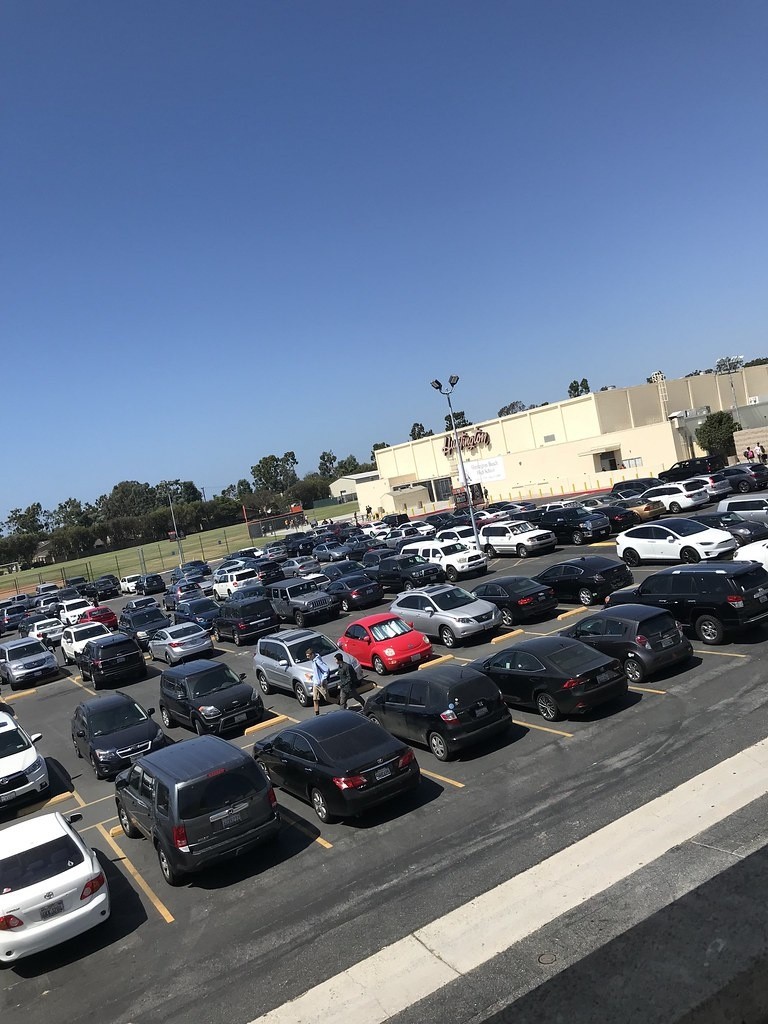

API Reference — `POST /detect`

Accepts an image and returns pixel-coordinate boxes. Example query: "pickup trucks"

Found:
[362,554,446,593]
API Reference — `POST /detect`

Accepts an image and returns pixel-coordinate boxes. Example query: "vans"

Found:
[717,493,768,524]
[399,538,487,582]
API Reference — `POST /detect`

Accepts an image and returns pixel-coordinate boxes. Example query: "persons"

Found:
[742,442,768,463]
[304,646,364,719]
[601,465,607,471]
[483,487,489,501]
[617,463,626,469]
[258,503,373,537]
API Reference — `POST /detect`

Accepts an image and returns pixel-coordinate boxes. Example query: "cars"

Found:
[586,506,639,533]
[0,636,60,692]
[469,575,559,625]
[146,622,214,667]
[0,452,729,666]
[731,539,768,573]
[614,517,739,565]
[530,554,635,607]
[463,635,629,722]
[327,574,385,613]
[361,664,513,762]
[555,602,694,683]
[252,709,426,824]
[336,612,434,675]
[685,471,733,503]
[636,480,711,514]
[0,710,50,813]
[0,812,112,973]
[613,496,666,525]
[387,583,504,650]
[69,689,168,782]
[685,511,768,547]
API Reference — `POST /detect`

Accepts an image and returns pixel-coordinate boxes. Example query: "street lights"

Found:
[155,478,186,563]
[429,374,484,551]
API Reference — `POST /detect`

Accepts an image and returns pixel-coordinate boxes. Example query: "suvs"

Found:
[604,560,768,645]
[477,520,559,559]
[252,626,362,708]
[539,506,610,546]
[211,594,281,647]
[266,578,339,628]
[715,462,768,496]
[76,633,147,692]
[159,659,264,738]
[115,733,284,883]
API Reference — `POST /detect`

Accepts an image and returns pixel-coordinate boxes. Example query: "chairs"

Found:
[115,712,131,724]
[51,848,73,862]
[39,627,44,630]
[53,623,57,626]
[28,860,44,870]
[47,625,50,628]
[440,596,450,607]
[94,722,108,732]
[191,585,196,589]
[489,586,497,592]
[0,867,23,883]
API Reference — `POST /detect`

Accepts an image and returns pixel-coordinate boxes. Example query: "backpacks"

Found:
[348,664,361,689]
